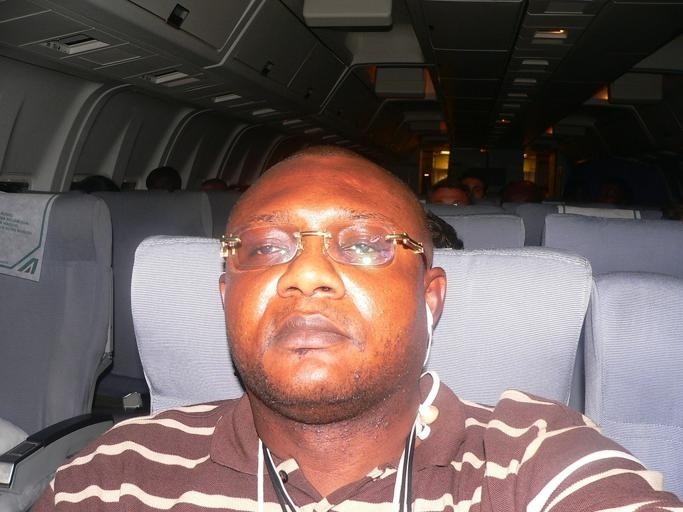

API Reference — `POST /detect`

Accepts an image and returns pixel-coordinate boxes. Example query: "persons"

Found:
[78,175,121,193]
[24,145,681,511]
[456,172,489,198]
[199,177,229,191]
[424,209,464,250]
[500,179,544,203]
[144,167,182,191]
[425,177,473,207]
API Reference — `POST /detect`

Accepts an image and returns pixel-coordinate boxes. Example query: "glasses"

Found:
[221,221,430,275]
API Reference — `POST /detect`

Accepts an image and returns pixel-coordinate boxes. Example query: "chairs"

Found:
[91,185,212,424]
[127,234,594,418]
[213,188,681,278]
[581,270,682,507]
[1,191,115,512]
